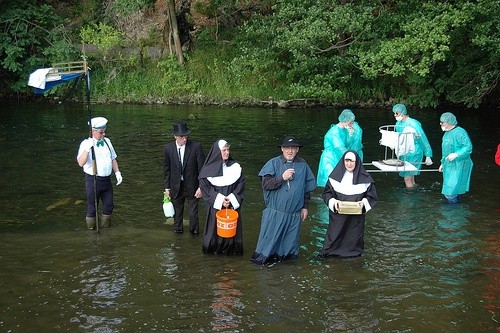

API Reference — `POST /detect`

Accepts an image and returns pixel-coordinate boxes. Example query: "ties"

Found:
[178,148,183,176]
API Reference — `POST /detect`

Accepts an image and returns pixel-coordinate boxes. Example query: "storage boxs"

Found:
[338,201,362,216]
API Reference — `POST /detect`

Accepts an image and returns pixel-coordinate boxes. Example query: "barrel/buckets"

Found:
[216,206,238,238]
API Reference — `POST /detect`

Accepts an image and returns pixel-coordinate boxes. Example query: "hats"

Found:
[88,117,108,131]
[276,135,304,149]
[170,120,191,136]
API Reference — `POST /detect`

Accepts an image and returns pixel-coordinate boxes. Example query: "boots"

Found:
[86,217,99,230]
[100,214,113,228]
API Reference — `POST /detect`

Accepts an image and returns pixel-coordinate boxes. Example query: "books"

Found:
[337,201,362,214]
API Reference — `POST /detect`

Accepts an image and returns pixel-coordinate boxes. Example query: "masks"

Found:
[395,115,404,121]
[344,123,354,136]
[441,125,450,131]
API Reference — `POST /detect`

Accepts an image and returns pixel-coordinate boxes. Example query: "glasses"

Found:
[442,122,444,124]
[176,135,189,140]
[393,112,398,115]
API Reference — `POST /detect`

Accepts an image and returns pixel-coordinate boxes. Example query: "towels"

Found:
[399,127,416,156]
[27,68,61,89]
[378,130,398,150]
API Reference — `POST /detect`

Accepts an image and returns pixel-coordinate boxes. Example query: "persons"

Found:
[77,117,123,230]
[439,112,473,201]
[318,150,379,257]
[198,140,247,253]
[250,137,316,264]
[161,121,205,233]
[393,104,433,187]
[495,144,500,166]
[316,109,364,186]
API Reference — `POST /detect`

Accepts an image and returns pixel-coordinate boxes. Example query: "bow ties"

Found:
[96,140,104,147]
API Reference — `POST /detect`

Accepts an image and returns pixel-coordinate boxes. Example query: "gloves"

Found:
[438,164,443,172]
[84,137,95,152]
[446,152,458,161]
[425,157,432,166]
[115,171,123,185]
